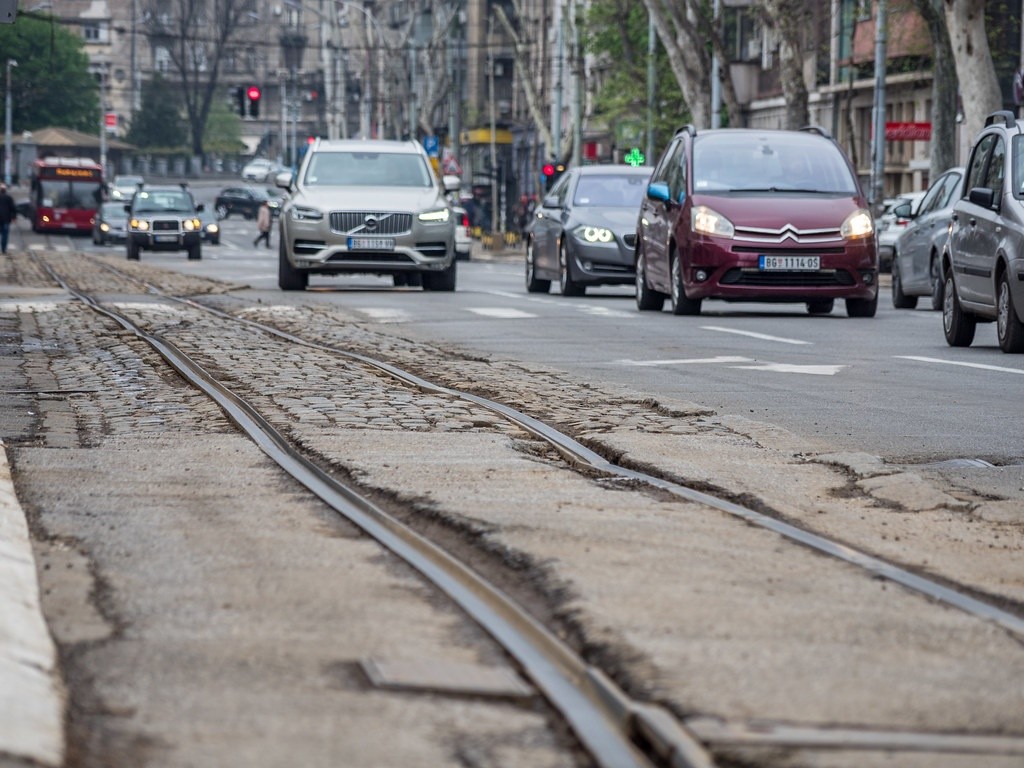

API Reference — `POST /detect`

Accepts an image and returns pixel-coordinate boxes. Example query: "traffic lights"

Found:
[247,85,262,118]
[228,85,246,117]
[544,162,565,193]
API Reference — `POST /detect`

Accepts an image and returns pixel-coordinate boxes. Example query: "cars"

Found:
[875,189,926,273]
[242,158,278,184]
[194,201,224,246]
[452,205,472,262]
[107,173,145,201]
[91,200,131,245]
[522,162,655,297]
[890,166,965,310]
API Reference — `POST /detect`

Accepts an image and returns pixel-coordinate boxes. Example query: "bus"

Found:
[25,155,110,236]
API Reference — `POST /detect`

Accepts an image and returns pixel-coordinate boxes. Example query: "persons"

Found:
[253,200,273,249]
[0,183,17,254]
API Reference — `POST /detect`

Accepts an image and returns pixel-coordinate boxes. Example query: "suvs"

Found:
[274,135,463,293]
[214,184,289,221]
[122,179,205,260]
[939,109,1024,354]
[632,123,883,319]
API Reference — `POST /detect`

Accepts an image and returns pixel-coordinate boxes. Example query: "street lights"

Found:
[4,57,20,186]
